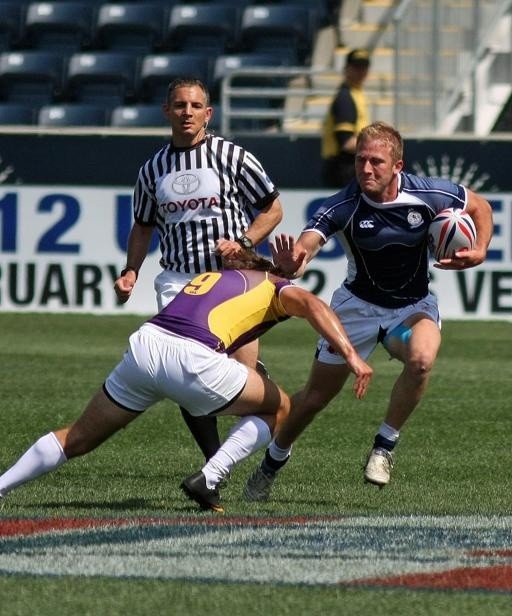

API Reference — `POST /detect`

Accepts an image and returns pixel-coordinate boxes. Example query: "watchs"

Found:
[235,237,253,250]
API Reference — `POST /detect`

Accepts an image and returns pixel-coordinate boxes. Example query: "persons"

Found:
[114,77,284,463]
[241,122,493,503]
[0,250,374,514]
[320,49,372,184]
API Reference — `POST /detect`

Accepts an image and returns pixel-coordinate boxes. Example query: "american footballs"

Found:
[426,206,477,264]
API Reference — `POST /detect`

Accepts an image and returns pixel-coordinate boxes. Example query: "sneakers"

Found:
[180,469,231,512]
[242,459,276,502]
[365,445,393,484]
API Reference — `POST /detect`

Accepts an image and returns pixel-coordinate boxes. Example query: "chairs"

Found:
[1,0,335,137]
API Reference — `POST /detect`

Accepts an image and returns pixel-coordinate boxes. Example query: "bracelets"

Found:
[121,267,138,280]
